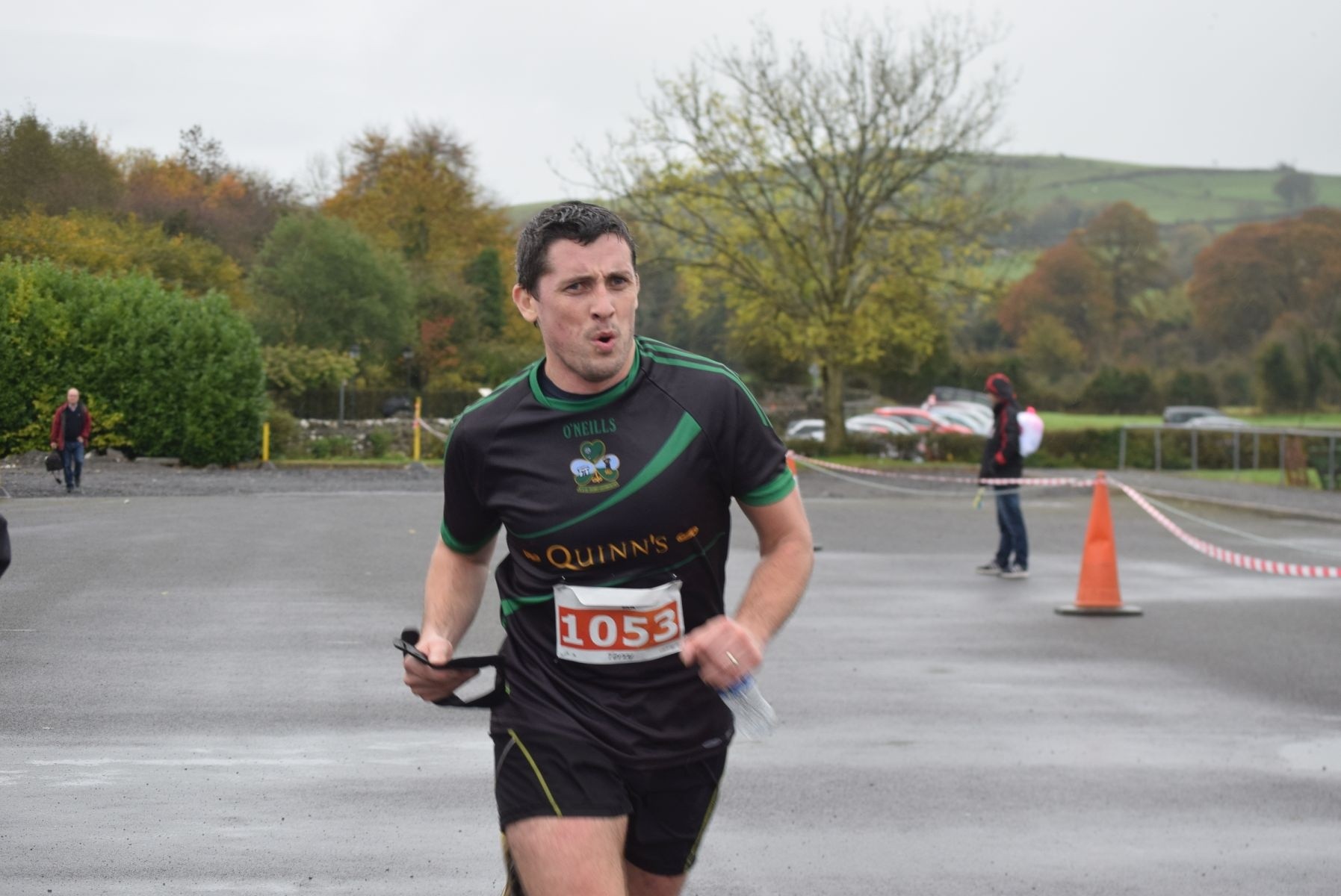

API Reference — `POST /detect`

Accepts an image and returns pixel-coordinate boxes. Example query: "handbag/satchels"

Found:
[45,449,64,484]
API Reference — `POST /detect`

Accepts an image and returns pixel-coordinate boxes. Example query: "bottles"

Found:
[719,672,780,738]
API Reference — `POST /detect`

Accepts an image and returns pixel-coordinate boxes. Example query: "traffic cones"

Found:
[1054,470,1145,618]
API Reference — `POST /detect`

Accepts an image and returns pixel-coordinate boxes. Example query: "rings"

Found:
[726,653,741,668]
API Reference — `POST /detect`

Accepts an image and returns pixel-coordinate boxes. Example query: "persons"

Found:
[406,199,818,896]
[52,387,90,492]
[979,374,1031,581]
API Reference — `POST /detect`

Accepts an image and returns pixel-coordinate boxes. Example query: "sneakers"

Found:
[974,561,1008,576]
[1000,563,1030,578]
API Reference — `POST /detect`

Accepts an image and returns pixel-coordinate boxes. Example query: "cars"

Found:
[1163,404,1248,429]
[783,382,1036,439]
